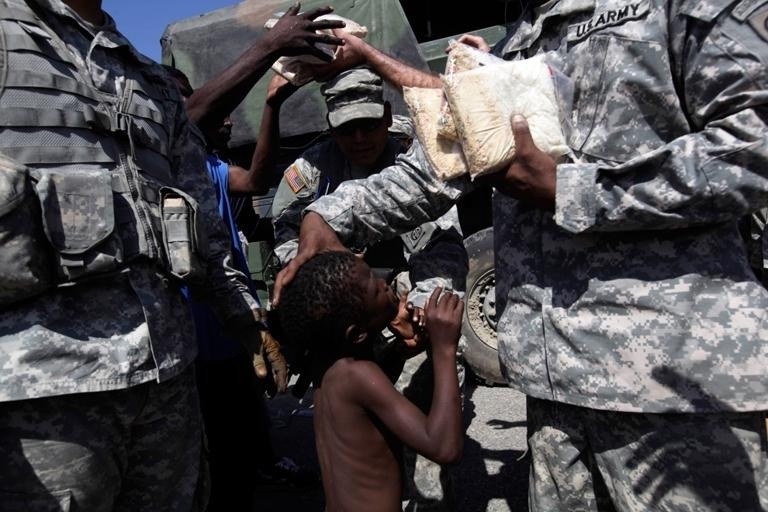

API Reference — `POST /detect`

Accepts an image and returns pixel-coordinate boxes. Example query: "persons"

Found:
[1,1,767,511]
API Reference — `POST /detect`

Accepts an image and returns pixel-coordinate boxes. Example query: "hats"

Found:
[317,66,385,128]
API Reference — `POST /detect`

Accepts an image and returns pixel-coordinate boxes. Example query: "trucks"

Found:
[159,1,573,398]
